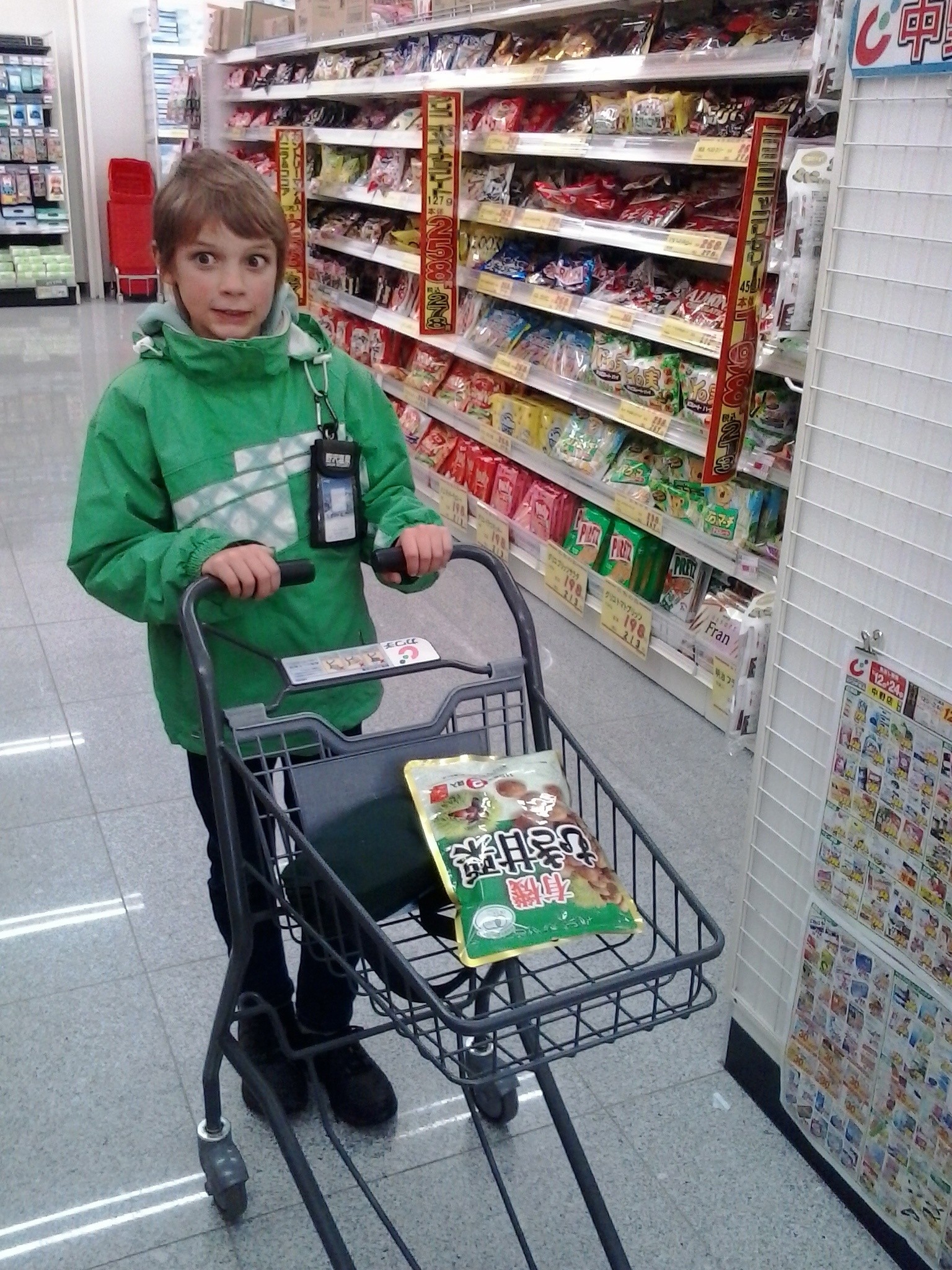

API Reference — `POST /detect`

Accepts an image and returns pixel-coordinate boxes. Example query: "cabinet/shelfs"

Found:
[371,0,836,733]
[188,32,377,367]
[0,28,81,308]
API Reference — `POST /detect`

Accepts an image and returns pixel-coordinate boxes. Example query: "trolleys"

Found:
[180,538,728,1270]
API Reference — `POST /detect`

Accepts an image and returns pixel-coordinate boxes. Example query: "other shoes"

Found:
[301,1025,399,1126]
[238,1000,309,1116]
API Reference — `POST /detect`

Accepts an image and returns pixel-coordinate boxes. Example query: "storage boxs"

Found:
[211,0,524,51]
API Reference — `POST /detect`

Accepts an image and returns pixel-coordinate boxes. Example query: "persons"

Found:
[68,145,454,1125]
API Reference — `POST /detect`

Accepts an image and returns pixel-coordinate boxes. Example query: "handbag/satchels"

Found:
[279,730,487,938]
